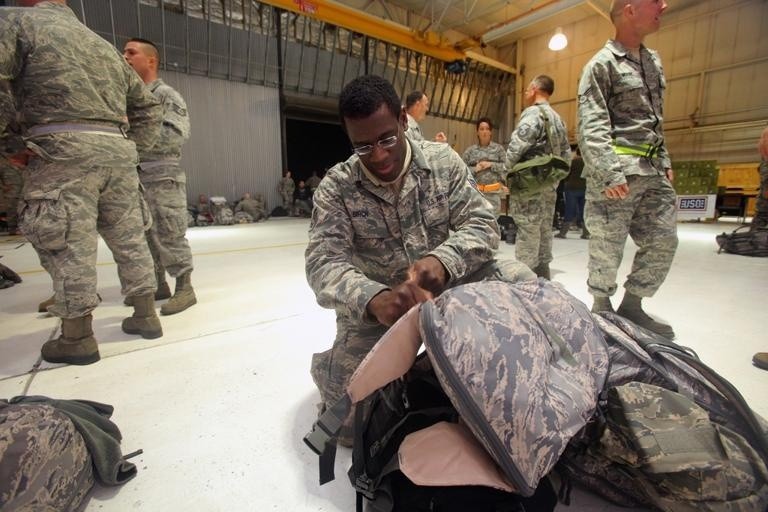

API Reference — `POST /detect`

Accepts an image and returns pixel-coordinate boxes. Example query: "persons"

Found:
[36,288,103,313]
[186,192,268,228]
[575,1,687,342]
[2,1,171,366]
[402,86,448,144]
[497,122,768,240]
[460,112,510,223]
[498,74,569,283]
[277,171,322,217]
[122,35,196,315]
[751,348,768,373]
[305,71,544,450]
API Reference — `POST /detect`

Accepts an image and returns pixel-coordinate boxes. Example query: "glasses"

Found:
[349,123,400,157]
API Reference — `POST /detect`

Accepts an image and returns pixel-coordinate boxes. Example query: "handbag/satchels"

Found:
[505,155,572,200]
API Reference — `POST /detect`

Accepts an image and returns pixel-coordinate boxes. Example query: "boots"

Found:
[590,291,675,339]
[38,269,197,364]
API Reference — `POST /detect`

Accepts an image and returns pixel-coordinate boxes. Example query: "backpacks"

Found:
[304,277,768,511]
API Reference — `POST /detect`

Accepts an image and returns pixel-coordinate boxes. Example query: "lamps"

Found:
[546,26,569,51]
